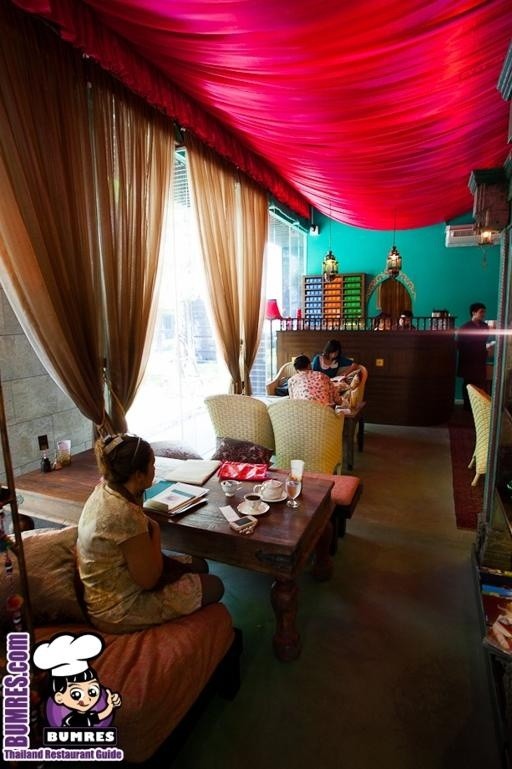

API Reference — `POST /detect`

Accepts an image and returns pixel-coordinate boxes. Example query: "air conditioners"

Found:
[445,223,500,248]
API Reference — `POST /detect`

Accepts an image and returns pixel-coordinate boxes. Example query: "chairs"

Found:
[334,364,370,403]
[204,393,275,451]
[463,384,493,482]
[266,397,344,475]
[265,362,310,401]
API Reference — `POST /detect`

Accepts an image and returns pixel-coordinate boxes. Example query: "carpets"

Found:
[449,423,484,531]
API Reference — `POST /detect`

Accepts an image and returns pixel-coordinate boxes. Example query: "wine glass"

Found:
[285,478,301,509]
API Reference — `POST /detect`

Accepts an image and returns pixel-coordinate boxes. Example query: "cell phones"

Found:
[229,515,258,531]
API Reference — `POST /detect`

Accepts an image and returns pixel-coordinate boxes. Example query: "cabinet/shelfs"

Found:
[303,273,367,329]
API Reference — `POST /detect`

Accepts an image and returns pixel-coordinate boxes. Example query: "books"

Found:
[148,481,208,519]
[164,457,222,485]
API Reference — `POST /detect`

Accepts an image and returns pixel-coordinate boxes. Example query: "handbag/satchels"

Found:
[218,460,277,481]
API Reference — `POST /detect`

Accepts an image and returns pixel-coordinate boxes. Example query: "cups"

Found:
[58,449,71,464]
[286,472,303,493]
[221,479,243,497]
[243,494,262,510]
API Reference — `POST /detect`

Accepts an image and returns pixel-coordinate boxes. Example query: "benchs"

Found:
[274,470,364,540]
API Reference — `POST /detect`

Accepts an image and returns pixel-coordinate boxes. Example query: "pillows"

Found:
[210,436,275,465]
[147,438,203,460]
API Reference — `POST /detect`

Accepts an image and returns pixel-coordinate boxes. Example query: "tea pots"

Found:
[253,480,284,499]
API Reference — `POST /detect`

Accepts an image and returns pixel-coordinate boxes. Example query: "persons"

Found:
[312,338,355,379]
[75,431,225,636]
[287,354,342,412]
[395,310,417,331]
[368,310,392,331]
[458,302,491,389]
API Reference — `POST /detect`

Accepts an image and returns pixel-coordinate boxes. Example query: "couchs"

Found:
[0,600,244,768]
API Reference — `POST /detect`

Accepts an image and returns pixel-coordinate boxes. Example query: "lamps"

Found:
[387,210,403,276]
[476,230,495,265]
[262,299,282,381]
[321,202,338,282]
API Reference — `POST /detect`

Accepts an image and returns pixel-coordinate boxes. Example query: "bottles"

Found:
[41,449,52,473]
[296,308,301,331]
[53,450,62,470]
[286,316,292,330]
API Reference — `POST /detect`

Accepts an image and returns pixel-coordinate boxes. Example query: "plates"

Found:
[237,502,270,515]
[263,491,287,503]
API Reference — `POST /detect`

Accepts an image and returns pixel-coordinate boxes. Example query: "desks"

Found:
[1,448,335,662]
[246,396,367,470]
